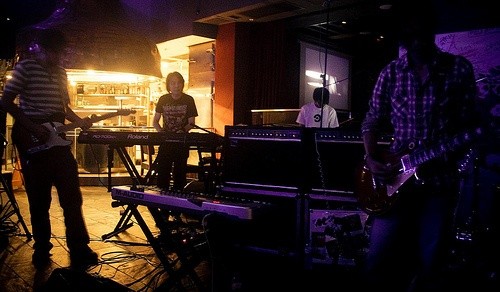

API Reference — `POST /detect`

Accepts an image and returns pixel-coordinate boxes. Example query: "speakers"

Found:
[219,125,395,195]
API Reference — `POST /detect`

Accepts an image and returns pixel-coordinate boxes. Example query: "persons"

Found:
[0,28,98,266]
[296,87,340,128]
[360,0,500,271]
[154,72,198,221]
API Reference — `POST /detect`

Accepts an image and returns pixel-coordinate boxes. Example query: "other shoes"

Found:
[32,251,51,269]
[175,217,182,224]
[157,220,169,228]
[69,245,98,263]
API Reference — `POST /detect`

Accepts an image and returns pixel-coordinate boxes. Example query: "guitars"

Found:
[12,109,139,159]
[354,120,500,215]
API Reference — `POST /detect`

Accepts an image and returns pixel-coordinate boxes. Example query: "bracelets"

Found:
[363,154,374,162]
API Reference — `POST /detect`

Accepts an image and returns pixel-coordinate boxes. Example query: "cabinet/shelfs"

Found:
[73,80,150,126]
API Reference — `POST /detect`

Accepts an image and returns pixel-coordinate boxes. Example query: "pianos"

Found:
[110,185,274,292]
[77,127,219,239]
